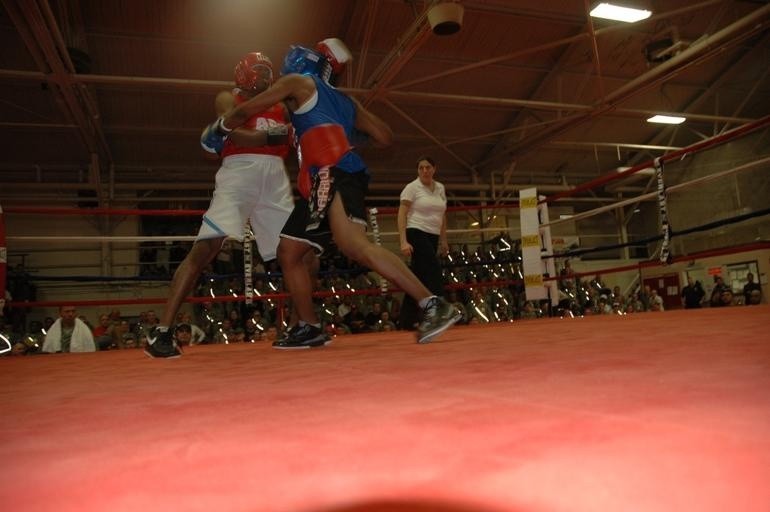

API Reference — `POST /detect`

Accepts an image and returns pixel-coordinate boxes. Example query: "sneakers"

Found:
[144,326,183,361]
[417,296,463,344]
[271,320,332,350]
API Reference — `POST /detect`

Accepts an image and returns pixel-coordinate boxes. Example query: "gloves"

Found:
[314,37,353,75]
[200,117,234,154]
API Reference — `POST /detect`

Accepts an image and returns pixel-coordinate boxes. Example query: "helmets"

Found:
[233,51,274,90]
[279,45,325,76]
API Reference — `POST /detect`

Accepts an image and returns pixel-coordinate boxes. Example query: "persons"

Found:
[2,263,208,356]
[681,272,762,309]
[143,39,464,357]
[142,237,662,344]
